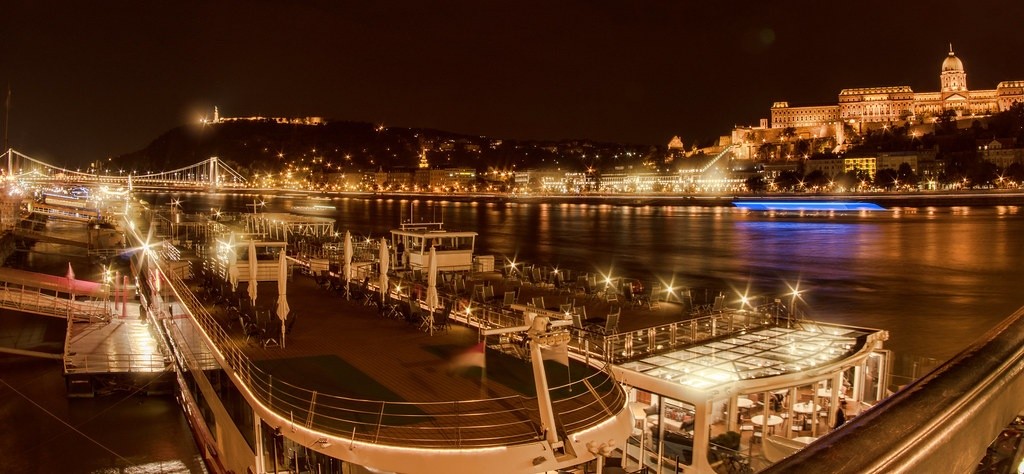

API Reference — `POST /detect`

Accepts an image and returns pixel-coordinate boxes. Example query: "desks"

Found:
[692,298,714,317]
[562,279,576,294]
[750,415,784,444]
[545,307,560,312]
[634,290,649,309]
[488,295,504,313]
[722,398,754,425]
[582,316,606,341]
[817,389,831,406]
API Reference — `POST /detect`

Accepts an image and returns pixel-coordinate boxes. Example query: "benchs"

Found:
[158,237,181,263]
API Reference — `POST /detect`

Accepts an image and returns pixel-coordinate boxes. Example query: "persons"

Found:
[774,394,783,412]
[679,412,694,433]
[833,401,847,429]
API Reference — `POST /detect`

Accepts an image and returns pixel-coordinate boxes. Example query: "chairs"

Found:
[294,234,600,330]
[570,313,593,344]
[198,268,296,351]
[752,424,762,443]
[793,403,822,426]
[739,415,754,442]
[792,419,803,432]
[796,399,808,420]
[818,406,830,426]
[595,314,620,345]
[604,277,726,320]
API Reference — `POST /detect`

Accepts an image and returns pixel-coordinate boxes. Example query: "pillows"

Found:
[643,403,657,418]
[679,418,693,432]
